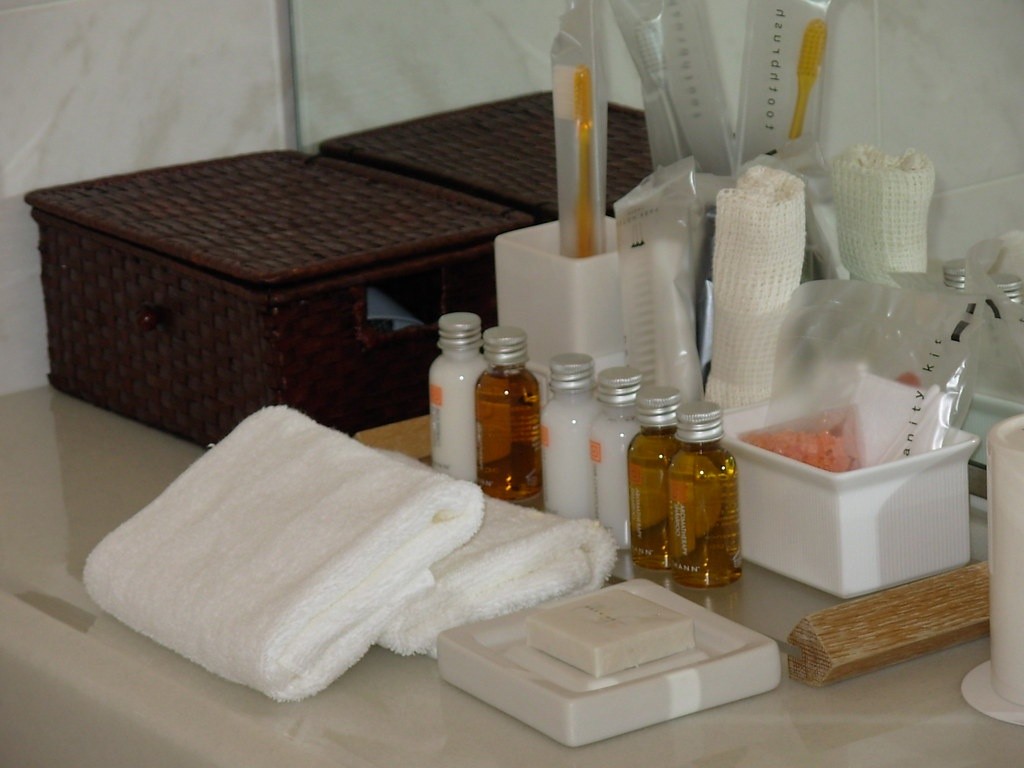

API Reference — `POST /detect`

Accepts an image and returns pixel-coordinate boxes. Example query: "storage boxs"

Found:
[316,89,654,222]
[22,148,543,449]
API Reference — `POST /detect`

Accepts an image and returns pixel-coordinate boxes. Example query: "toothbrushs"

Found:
[786,19,824,138]
[548,62,595,257]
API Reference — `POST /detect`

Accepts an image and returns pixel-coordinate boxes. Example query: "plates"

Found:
[961,660,1024,726]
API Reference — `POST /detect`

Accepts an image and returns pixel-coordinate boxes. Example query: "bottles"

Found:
[593,366,646,550]
[626,388,683,571]
[992,274,1024,305]
[668,400,742,588]
[473,326,542,504]
[426,311,486,482]
[541,352,598,521]
[941,258,972,290]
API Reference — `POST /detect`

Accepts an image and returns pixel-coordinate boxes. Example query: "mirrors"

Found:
[286,0,1024,466]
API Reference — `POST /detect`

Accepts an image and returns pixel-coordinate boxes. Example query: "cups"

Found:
[493,213,628,364]
[985,413,1024,705]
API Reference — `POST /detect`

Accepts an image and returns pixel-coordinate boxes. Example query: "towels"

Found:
[79,402,486,702]
[374,494,618,657]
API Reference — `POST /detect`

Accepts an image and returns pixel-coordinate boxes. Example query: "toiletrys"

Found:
[624,385,678,584]
[591,364,645,553]
[540,353,594,513]
[667,401,744,591]
[474,324,540,504]
[426,311,481,482]
